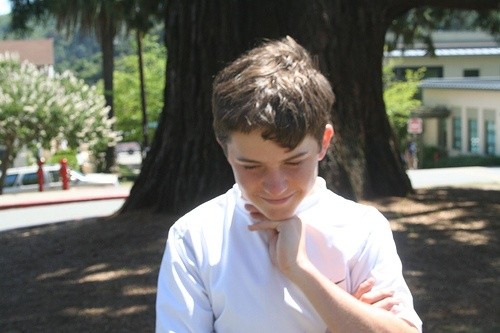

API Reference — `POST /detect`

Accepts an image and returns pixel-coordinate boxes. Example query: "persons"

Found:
[155,35,424,333]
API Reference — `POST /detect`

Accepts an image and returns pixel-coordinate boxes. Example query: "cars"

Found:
[0,166,118,196]
